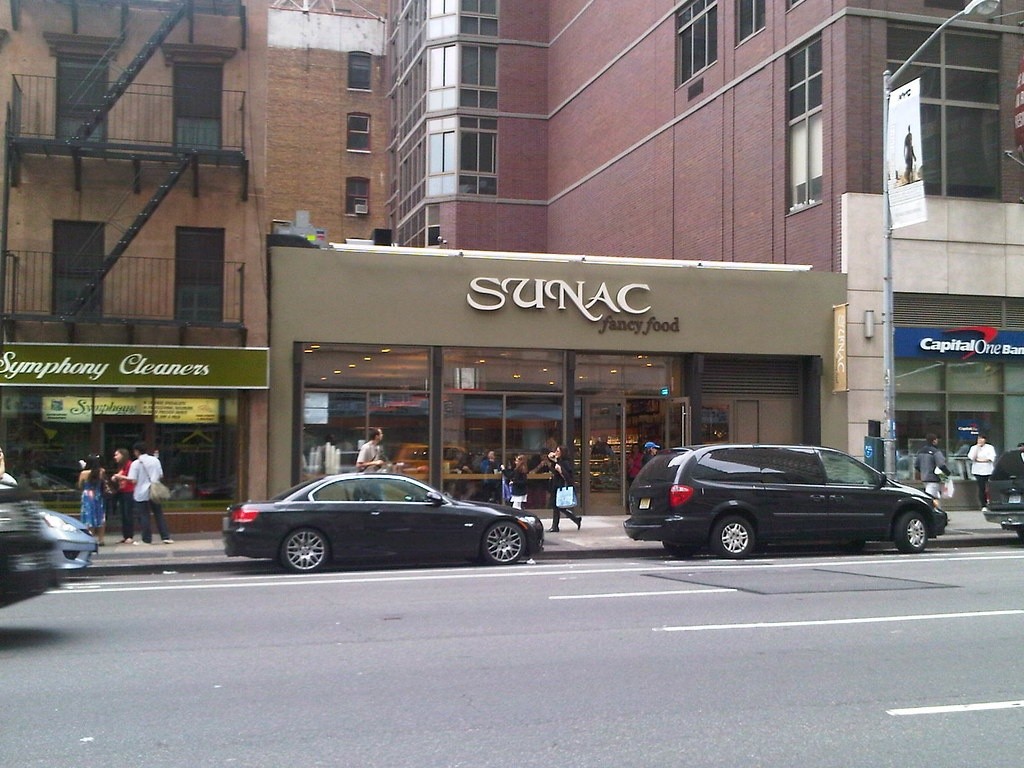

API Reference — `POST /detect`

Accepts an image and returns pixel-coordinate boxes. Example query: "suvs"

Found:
[622,442,950,559]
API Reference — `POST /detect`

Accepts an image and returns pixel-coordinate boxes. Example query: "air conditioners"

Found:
[354,204,368,215]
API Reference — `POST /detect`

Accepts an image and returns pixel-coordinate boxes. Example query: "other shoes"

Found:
[115,537,133,544]
[546,528,558,532]
[982,507,987,512]
[578,517,581,530]
[97,540,105,546]
[133,540,151,545]
[162,538,174,543]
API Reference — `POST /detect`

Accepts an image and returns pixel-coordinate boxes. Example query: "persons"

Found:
[627,439,660,483]
[967,434,996,512]
[545,445,581,531]
[481,450,497,474]
[500,454,528,508]
[915,433,950,498]
[354,427,406,500]
[114,442,173,544]
[327,432,353,450]
[591,437,614,456]
[111,448,134,543]
[78,453,106,547]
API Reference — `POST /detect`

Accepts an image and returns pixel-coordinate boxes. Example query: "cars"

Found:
[983,442,1024,543]
[1,481,99,609]
[220,471,544,574]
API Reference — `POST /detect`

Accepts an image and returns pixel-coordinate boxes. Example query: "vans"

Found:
[393,441,469,485]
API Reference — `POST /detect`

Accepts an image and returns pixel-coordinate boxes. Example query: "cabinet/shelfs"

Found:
[591,404,665,477]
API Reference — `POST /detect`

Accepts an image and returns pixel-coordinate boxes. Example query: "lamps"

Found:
[33,420,58,438]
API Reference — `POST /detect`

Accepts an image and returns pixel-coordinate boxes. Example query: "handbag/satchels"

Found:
[943,479,954,499]
[556,479,577,509]
[150,481,171,504]
[103,479,117,500]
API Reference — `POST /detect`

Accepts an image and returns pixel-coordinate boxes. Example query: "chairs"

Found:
[352,480,385,502]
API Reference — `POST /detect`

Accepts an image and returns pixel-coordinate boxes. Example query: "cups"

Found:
[397,463,404,473]
[493,469,498,474]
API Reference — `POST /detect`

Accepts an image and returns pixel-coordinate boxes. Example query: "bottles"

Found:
[387,462,397,473]
[507,460,511,471]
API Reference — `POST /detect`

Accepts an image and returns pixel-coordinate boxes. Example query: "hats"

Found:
[644,442,660,448]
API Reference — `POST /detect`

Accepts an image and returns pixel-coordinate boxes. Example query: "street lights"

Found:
[881,0,1003,484]
